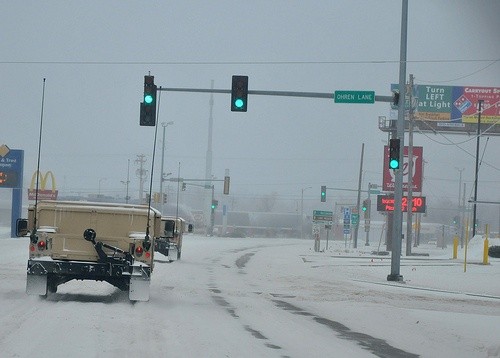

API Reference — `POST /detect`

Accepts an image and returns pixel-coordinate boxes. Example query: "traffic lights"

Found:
[475,219,479,228]
[362,201,370,219]
[211,201,217,212]
[389,138,401,170]
[140,103,156,126]
[145,193,149,203]
[144,75,155,108]
[182,183,187,191]
[152,192,158,203]
[230,75,248,113]
[453,217,459,225]
[320,185,327,202]
[163,193,167,204]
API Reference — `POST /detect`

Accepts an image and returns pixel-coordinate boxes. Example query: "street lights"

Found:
[300,187,312,218]
[160,121,174,214]
[473,98,484,236]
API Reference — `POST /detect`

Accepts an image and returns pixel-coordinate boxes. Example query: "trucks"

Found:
[15,201,193,303]
